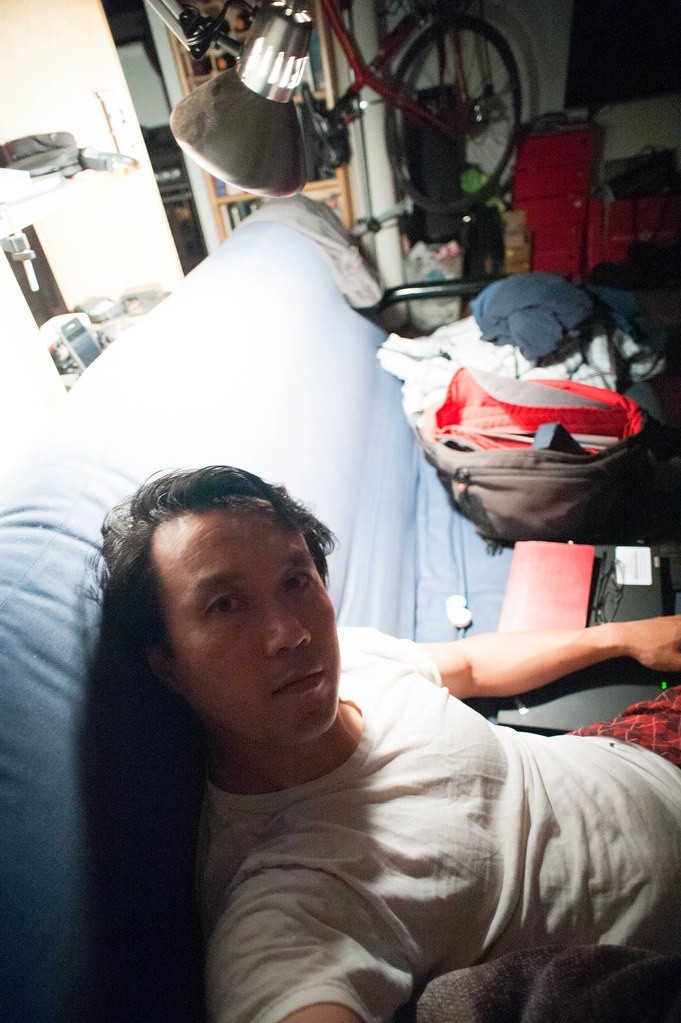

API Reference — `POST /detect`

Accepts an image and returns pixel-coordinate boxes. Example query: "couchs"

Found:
[0,199,660,1023]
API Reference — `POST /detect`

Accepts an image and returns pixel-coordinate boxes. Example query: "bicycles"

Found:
[179,0,524,220]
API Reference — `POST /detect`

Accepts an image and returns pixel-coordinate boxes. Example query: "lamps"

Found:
[144,0,317,198]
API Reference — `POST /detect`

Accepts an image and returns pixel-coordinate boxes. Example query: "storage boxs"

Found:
[510,128,597,275]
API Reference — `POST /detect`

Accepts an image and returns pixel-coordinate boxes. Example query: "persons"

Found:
[97,464,680,1023]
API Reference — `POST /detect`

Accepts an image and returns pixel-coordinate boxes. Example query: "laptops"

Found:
[497,542,665,742]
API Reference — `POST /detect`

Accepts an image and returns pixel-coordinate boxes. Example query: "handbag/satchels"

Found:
[420,380,649,543]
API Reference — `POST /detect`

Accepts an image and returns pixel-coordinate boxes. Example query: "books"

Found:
[213,176,261,237]
